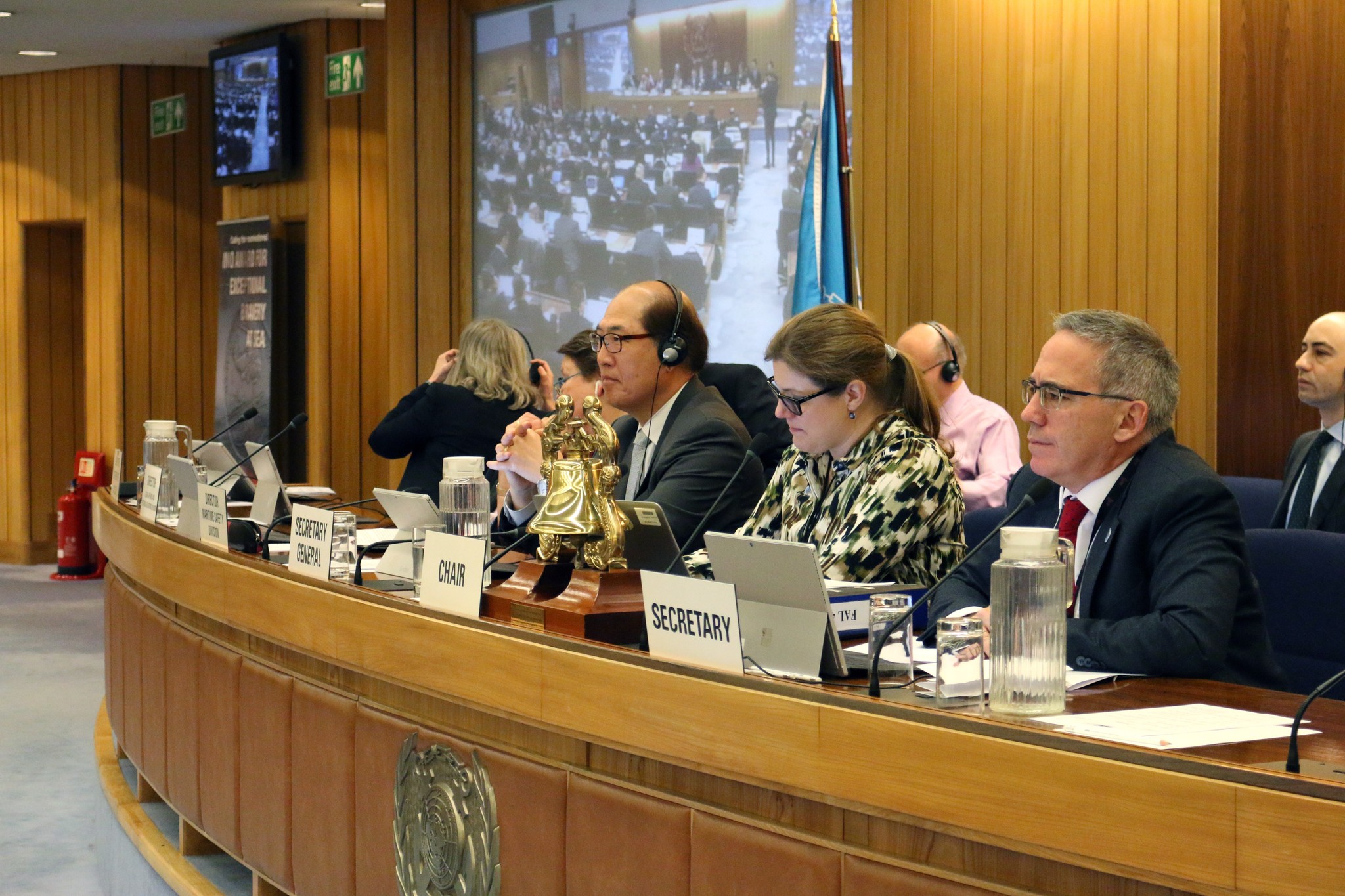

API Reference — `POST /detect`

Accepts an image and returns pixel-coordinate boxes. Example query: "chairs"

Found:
[1224,476,1345,704]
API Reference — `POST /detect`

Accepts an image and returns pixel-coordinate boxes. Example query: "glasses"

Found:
[588,332,663,353]
[767,376,847,415]
[553,372,582,395]
[1020,379,1134,411]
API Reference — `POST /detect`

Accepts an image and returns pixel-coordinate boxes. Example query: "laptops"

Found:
[185,440,257,502]
[701,530,933,678]
[244,442,381,525]
[372,488,519,578]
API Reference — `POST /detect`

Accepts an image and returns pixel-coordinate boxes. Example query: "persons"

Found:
[1269,311,1345,532]
[474,92,739,389]
[368,318,558,510]
[781,99,853,213]
[787,229,799,250]
[922,309,1285,688]
[623,56,778,90]
[896,321,1023,513]
[682,303,968,583]
[215,80,281,176]
[486,279,765,558]
[490,330,628,546]
[758,68,777,168]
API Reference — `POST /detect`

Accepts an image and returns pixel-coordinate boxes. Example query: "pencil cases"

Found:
[227,519,263,554]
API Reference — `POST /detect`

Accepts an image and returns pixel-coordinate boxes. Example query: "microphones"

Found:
[639,430,769,653]
[182,406,258,458]
[868,476,1050,698]
[354,526,535,587]
[207,413,310,486]
[261,485,421,562]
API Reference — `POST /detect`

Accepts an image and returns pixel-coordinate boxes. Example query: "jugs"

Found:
[988,526,1076,717]
[142,419,193,521]
[439,456,492,588]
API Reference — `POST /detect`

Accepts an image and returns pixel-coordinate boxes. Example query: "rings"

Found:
[504,445,510,451]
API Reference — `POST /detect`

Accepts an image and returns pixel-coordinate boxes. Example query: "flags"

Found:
[792,30,862,317]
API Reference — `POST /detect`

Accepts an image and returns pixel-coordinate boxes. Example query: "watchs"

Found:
[496,483,508,497]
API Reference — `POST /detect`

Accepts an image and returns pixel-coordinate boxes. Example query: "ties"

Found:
[625,429,651,501]
[1288,431,1333,529]
[1058,496,1089,618]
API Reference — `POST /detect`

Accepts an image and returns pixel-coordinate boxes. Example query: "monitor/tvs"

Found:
[207,30,301,186]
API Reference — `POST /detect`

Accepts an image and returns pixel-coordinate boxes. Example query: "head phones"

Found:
[925,320,960,384]
[656,279,689,367]
[510,324,542,384]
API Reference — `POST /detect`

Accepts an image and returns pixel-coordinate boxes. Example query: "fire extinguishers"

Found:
[57,477,89,574]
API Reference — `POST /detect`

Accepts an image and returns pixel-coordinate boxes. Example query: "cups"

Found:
[935,617,985,709]
[136,466,144,510]
[412,524,447,597]
[195,465,207,484]
[329,511,357,579]
[868,594,914,691]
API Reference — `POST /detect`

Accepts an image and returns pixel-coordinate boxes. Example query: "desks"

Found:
[88,475,1345,896]
